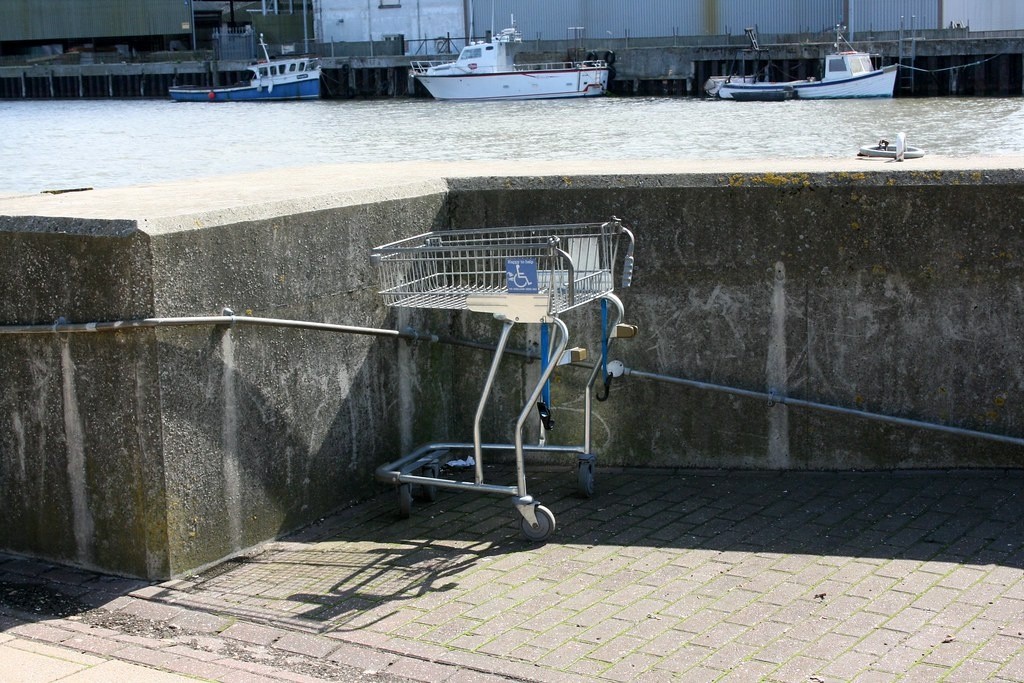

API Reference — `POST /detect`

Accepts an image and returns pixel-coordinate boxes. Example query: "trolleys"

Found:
[368,216,638,542]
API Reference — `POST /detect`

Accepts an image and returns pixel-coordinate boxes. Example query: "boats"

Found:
[408,13,609,102]
[717,26,901,101]
[167,34,324,102]
